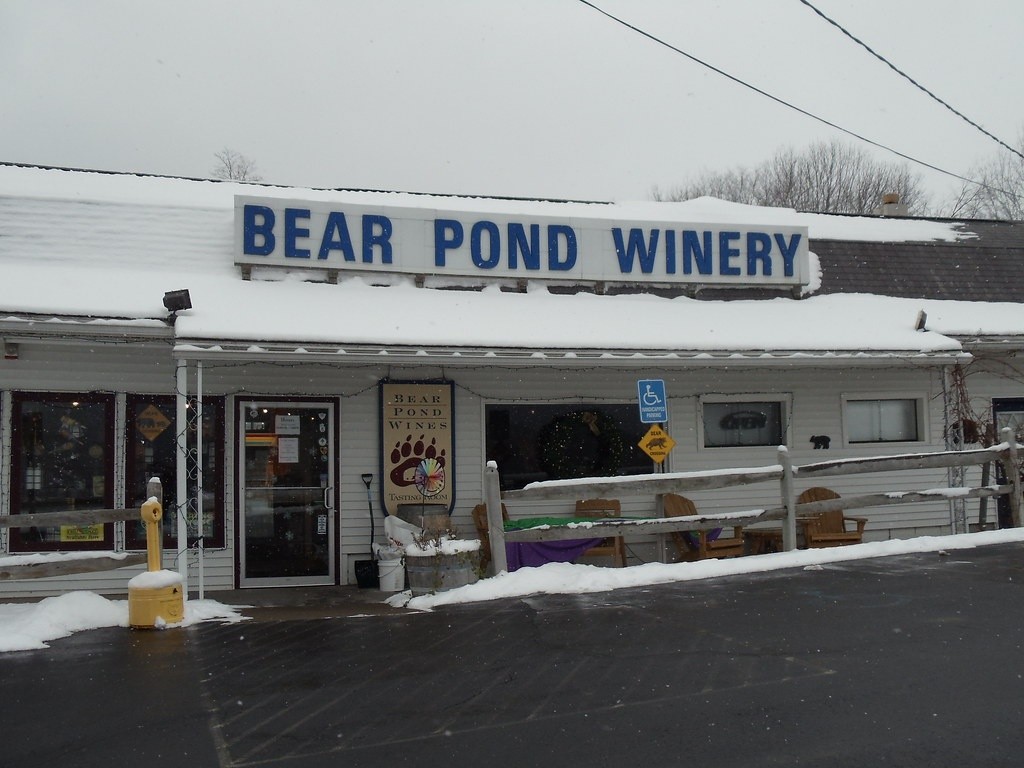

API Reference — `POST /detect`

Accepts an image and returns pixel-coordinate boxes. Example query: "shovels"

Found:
[354,473,381,589]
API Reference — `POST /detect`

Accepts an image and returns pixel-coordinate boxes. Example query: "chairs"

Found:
[657,493,745,564]
[798,487,868,549]
[472,503,512,578]
[574,499,628,568]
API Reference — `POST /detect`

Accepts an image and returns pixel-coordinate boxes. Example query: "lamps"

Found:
[163,289,193,327]
[915,309,931,333]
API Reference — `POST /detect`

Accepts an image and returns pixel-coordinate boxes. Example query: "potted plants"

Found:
[400,527,486,596]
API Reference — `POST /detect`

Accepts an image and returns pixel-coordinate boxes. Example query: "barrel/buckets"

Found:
[397,503,450,533]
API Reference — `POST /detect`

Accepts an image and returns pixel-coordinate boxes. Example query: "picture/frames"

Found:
[379,378,455,517]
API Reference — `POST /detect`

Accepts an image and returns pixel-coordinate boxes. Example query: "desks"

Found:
[743,526,803,556]
